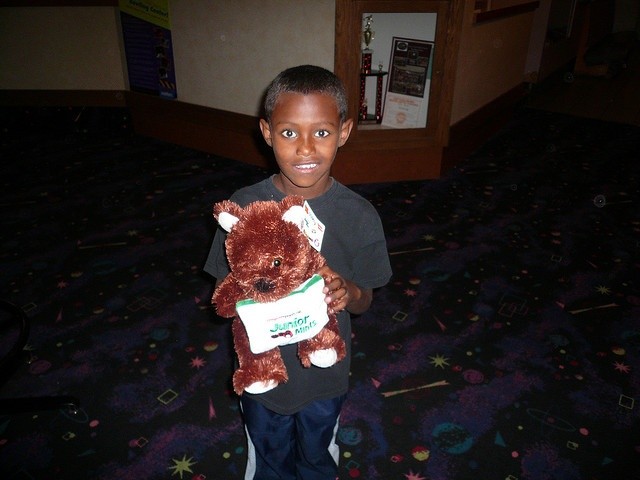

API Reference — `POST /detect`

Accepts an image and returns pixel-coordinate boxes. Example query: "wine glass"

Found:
[378,58,384,73]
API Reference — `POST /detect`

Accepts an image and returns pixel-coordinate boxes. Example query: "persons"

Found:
[203,65,393,480]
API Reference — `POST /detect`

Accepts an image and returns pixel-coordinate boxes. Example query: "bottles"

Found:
[362,104,367,121]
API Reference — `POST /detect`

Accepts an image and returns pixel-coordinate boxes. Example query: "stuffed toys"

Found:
[211,194,347,396]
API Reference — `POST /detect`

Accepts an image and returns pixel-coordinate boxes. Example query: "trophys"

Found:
[362,15,374,69]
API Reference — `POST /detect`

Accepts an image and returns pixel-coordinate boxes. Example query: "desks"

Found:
[358,70,387,123]
